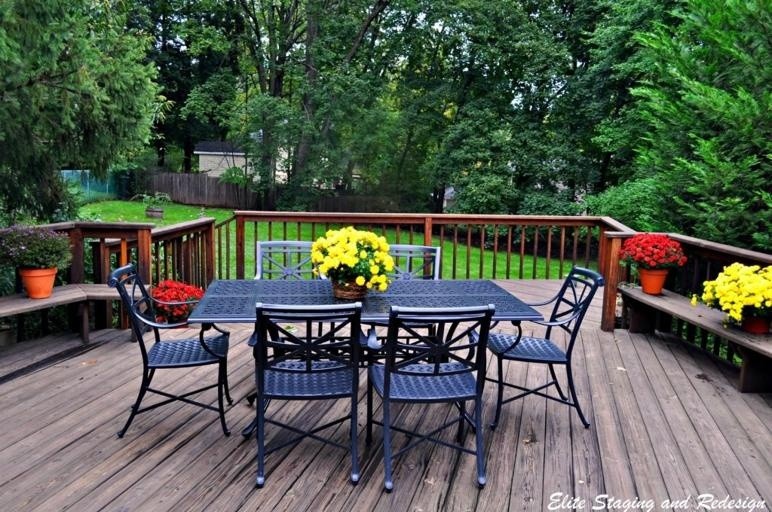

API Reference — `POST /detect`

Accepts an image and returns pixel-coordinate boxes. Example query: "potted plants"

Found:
[130,190,176,218]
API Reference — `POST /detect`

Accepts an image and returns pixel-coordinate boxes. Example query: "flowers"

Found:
[617,227,688,273]
[310,216,394,294]
[688,260,771,329]
[0,224,72,271]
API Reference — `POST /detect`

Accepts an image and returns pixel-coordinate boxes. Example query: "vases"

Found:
[745,308,772,336]
[636,266,669,295]
[16,264,60,300]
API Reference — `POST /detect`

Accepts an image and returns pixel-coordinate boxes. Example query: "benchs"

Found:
[0,283,90,387]
[616,283,772,393]
